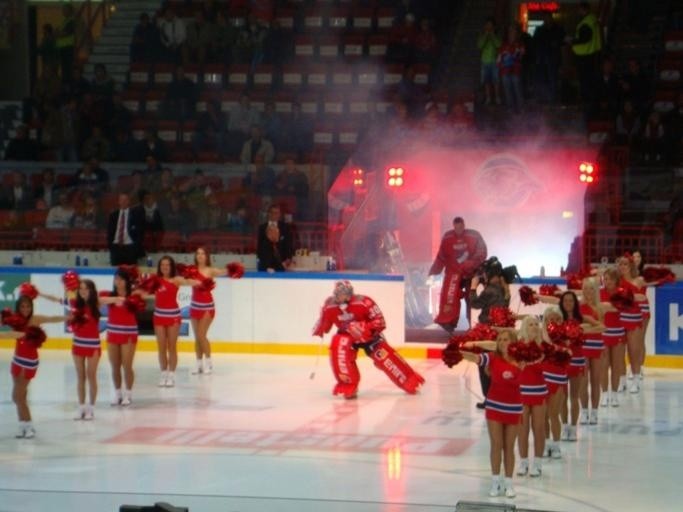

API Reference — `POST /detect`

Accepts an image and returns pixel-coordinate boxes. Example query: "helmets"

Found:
[333,280,353,304]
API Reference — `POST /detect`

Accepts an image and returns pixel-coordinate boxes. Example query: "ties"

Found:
[119,212,125,248]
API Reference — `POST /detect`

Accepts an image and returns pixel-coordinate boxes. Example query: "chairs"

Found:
[0,161,328,254]
[0,0,683,160]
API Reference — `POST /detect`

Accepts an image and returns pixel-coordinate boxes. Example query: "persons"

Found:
[173,247,242,374]
[95,263,157,407]
[391,13,438,63]
[1,293,77,439]
[427,217,487,334]
[0,330,27,339]
[35,278,127,422]
[310,279,426,399]
[443,252,674,497]
[370,239,394,271]
[388,101,472,144]
[475,3,665,163]
[133,256,204,388]
[2,2,307,272]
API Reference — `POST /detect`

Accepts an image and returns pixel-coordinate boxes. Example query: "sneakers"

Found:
[72,407,94,420]
[15,423,37,438]
[491,368,644,499]
[110,393,132,405]
[191,364,213,374]
[158,376,174,387]
